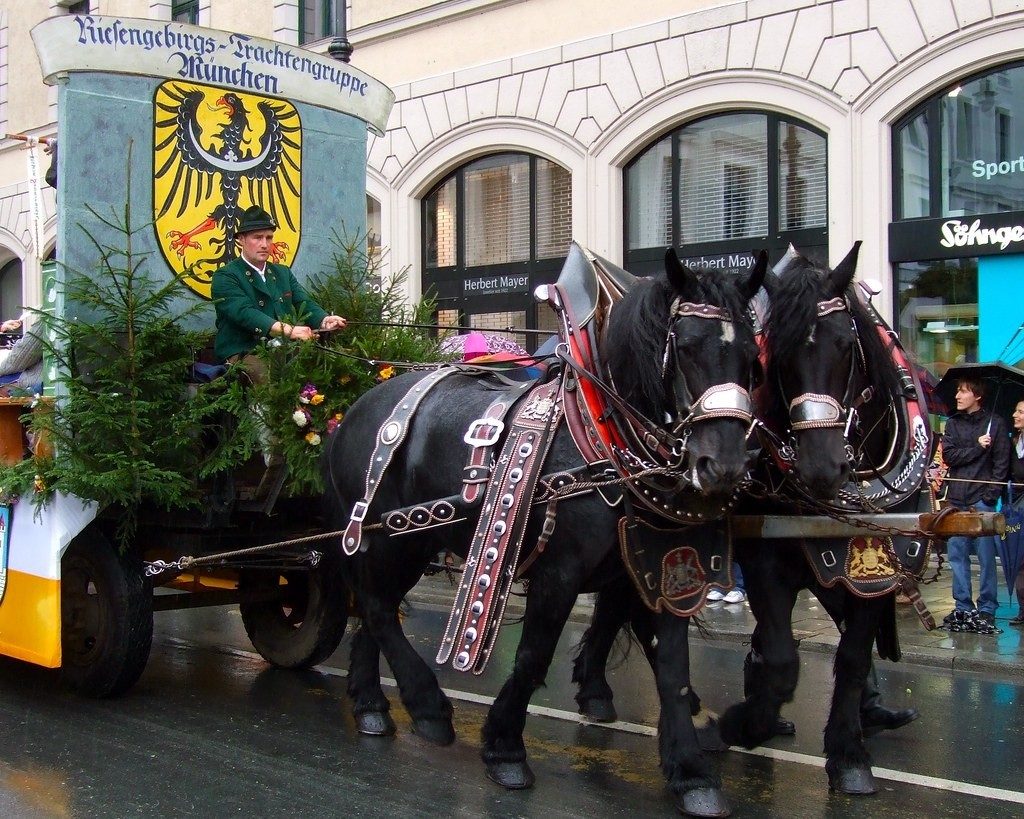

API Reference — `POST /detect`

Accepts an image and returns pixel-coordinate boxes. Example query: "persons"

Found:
[705,376,1024,631]
[0,308,43,387]
[210,204,348,468]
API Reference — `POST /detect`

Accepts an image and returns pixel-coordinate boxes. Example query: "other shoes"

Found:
[943,612,956,622]
[1010,617,1024,623]
[979,611,994,625]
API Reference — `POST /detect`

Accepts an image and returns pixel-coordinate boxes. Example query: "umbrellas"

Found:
[929,360,1024,447]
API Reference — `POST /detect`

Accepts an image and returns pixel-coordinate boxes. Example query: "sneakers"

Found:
[723,591,745,603]
[706,591,724,601]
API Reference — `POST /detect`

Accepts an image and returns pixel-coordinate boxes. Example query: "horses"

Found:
[305,238,953,819]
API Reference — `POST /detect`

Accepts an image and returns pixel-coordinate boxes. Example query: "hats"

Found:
[233,206,277,239]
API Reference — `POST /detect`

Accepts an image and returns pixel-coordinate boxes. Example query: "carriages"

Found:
[0,14,1005,817]
